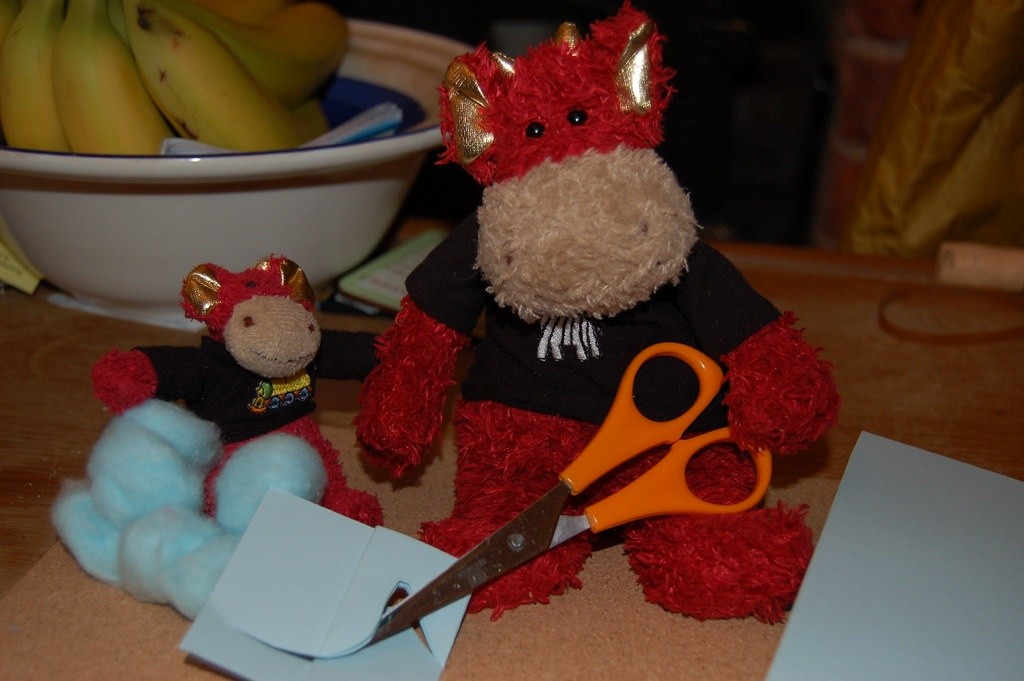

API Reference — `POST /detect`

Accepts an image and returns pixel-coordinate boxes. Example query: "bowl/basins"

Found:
[0,18,482,305]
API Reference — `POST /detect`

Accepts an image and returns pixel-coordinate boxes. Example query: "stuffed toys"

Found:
[350,0,842,624]
[89,255,385,529]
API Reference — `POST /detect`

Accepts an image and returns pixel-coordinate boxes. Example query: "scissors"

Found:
[364,340,777,648]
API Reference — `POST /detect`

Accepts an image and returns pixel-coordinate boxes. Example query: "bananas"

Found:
[0,0,352,155]
